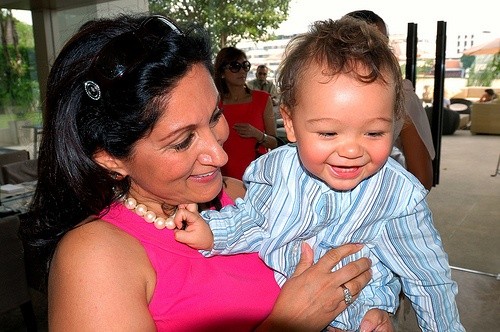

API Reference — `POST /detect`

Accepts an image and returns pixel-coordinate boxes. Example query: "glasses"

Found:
[256,72,267,75]
[80,15,184,103]
[225,61,251,73]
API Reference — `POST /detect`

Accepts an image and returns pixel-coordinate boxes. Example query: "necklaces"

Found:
[111,187,182,230]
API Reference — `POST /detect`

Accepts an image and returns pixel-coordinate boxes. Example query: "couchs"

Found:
[470,101,500,136]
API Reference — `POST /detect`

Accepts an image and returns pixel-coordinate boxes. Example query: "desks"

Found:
[22,124,43,160]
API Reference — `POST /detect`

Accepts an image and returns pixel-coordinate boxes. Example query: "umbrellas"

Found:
[462,36,500,56]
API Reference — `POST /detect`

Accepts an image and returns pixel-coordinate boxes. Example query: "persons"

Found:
[442,97,450,111]
[423,85,432,103]
[247,65,279,106]
[21,13,395,331]
[174,18,466,332]
[214,47,278,181]
[465,89,497,128]
[339,10,435,194]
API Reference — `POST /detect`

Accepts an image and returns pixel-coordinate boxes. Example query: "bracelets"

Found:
[259,134,268,143]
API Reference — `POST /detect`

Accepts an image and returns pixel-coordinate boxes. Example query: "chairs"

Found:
[0,217,39,331]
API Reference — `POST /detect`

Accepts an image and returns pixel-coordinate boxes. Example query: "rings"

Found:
[341,285,353,305]
